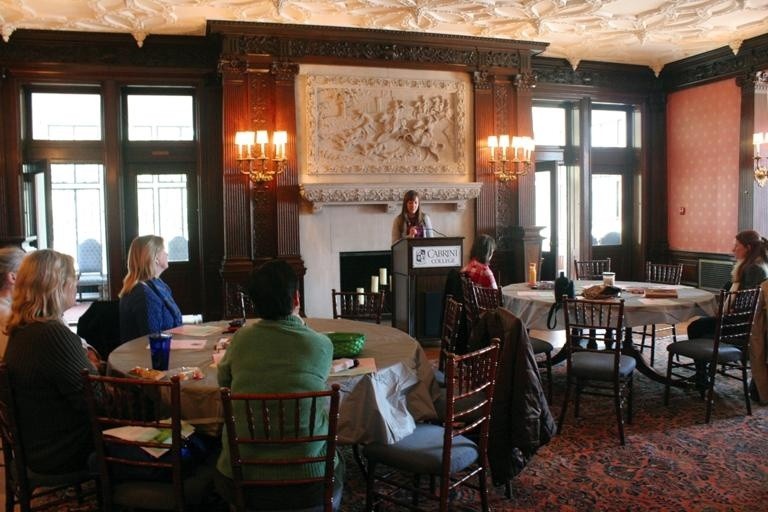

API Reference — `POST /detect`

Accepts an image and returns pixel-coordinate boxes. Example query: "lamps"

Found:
[484,135,537,187]
[235,129,292,186]
[750,132,768,186]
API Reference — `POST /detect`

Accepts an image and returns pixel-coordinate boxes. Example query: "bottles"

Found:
[555,271,569,302]
[528,263,537,286]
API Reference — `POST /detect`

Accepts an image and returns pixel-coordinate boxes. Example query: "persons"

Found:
[685,228,767,391]
[391,190,433,245]
[330,90,452,158]
[210,256,347,511]
[0,245,29,360]
[458,233,499,321]
[115,234,184,345]
[0,250,106,498]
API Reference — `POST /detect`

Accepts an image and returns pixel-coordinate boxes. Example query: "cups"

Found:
[148,334,171,370]
[602,272,615,286]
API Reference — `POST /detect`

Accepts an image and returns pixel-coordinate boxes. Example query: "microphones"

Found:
[415,228,446,237]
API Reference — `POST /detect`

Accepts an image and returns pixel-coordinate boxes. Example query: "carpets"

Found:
[338,335,768,512]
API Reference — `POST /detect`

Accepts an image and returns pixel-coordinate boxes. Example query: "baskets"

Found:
[324,330,365,359]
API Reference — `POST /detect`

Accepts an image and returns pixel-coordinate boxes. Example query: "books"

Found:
[99,421,162,447]
[140,415,196,460]
[641,286,679,300]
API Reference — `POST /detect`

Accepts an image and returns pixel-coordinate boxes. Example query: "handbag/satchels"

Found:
[553,271,576,303]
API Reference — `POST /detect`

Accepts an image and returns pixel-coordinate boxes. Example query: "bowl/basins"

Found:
[318,330,366,357]
